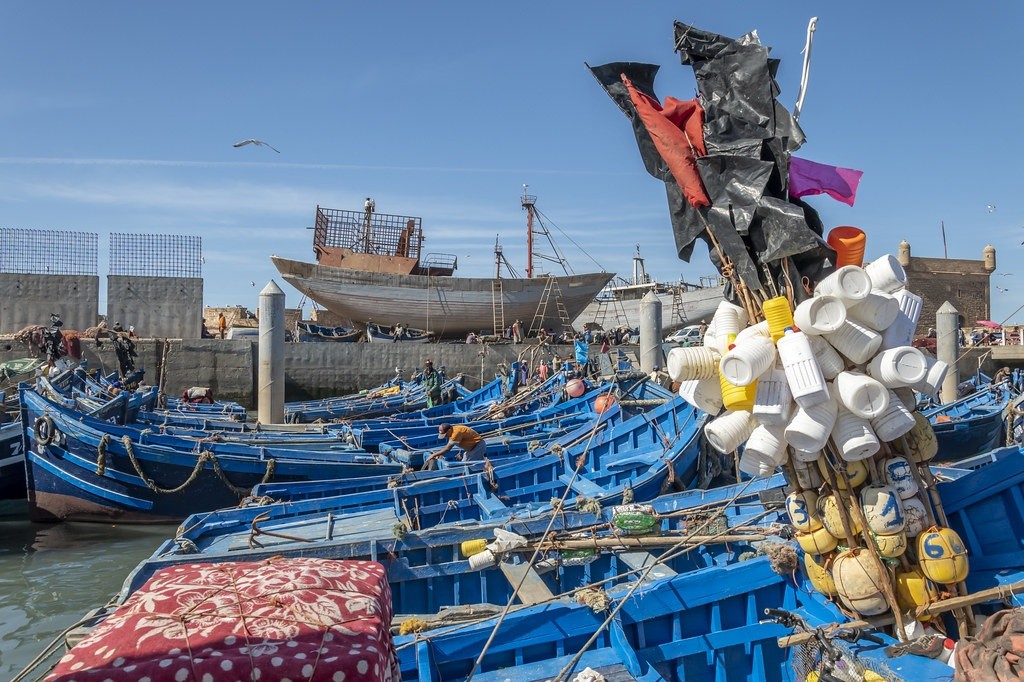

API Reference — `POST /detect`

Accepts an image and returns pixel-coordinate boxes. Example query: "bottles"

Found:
[922,621,958,669]
[666,225,948,478]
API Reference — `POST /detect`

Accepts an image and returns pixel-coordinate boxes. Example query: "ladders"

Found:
[491,281,505,337]
[594,280,631,329]
[670,291,689,333]
[527,275,575,339]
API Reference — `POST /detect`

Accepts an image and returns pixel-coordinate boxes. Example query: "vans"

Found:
[671,324,709,346]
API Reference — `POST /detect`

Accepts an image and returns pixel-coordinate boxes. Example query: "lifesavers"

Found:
[34,416,54,445]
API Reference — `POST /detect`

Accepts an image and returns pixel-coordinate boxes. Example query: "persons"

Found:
[927,328,936,337]
[113,321,123,332]
[364,198,371,219]
[411,359,441,407]
[202,318,215,339]
[960,328,989,346]
[995,367,1014,386]
[521,360,528,385]
[513,319,639,345]
[539,359,546,383]
[394,323,404,343]
[552,354,561,374]
[426,423,486,461]
[466,334,476,344]
[219,313,226,339]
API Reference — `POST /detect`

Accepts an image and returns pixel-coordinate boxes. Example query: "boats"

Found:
[570,244,728,339]
[0,357,1024,682]
[270,182,616,334]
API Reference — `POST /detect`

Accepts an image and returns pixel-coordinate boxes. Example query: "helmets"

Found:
[426,359,433,365]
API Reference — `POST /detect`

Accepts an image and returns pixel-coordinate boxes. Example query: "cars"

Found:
[665,329,681,343]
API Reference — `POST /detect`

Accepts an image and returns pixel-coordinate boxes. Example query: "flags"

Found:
[789,156,862,208]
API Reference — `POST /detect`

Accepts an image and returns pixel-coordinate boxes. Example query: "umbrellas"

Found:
[976,320,1004,332]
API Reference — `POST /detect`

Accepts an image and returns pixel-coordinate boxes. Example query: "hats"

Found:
[438,423,452,439]
[522,360,527,364]
